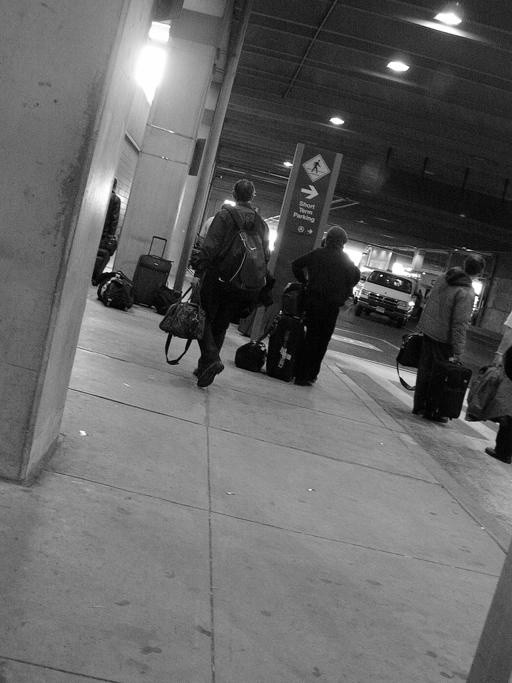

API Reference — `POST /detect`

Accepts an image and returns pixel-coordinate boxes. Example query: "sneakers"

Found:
[198,360,224,387]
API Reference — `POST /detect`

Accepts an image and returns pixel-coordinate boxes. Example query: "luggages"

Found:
[426,358,472,421]
[266,313,303,380]
[92,236,116,285]
[133,235,171,308]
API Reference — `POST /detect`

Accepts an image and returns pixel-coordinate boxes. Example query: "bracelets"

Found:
[493,351,504,356]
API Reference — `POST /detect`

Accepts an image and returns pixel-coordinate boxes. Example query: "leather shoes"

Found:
[486,447,511,463]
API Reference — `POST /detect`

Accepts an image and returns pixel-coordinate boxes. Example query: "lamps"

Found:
[386,51,411,72]
[433,0,467,27]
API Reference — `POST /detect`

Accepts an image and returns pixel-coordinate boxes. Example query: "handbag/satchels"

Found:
[396,332,424,368]
[100,238,118,256]
[149,285,182,315]
[282,289,306,314]
[235,341,266,371]
[97,274,134,311]
[466,366,512,421]
[159,286,205,341]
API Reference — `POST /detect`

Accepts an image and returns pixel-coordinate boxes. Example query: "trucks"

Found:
[407,270,440,313]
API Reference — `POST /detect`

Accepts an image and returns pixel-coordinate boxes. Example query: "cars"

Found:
[353,271,370,300]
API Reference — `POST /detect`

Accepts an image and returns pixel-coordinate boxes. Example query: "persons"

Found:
[291,225,362,387]
[91,176,120,284]
[484,311,512,461]
[192,176,270,387]
[411,252,487,423]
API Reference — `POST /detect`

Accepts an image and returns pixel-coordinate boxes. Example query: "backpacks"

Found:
[221,204,269,294]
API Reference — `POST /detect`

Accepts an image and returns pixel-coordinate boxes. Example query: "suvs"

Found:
[356,269,418,325]
